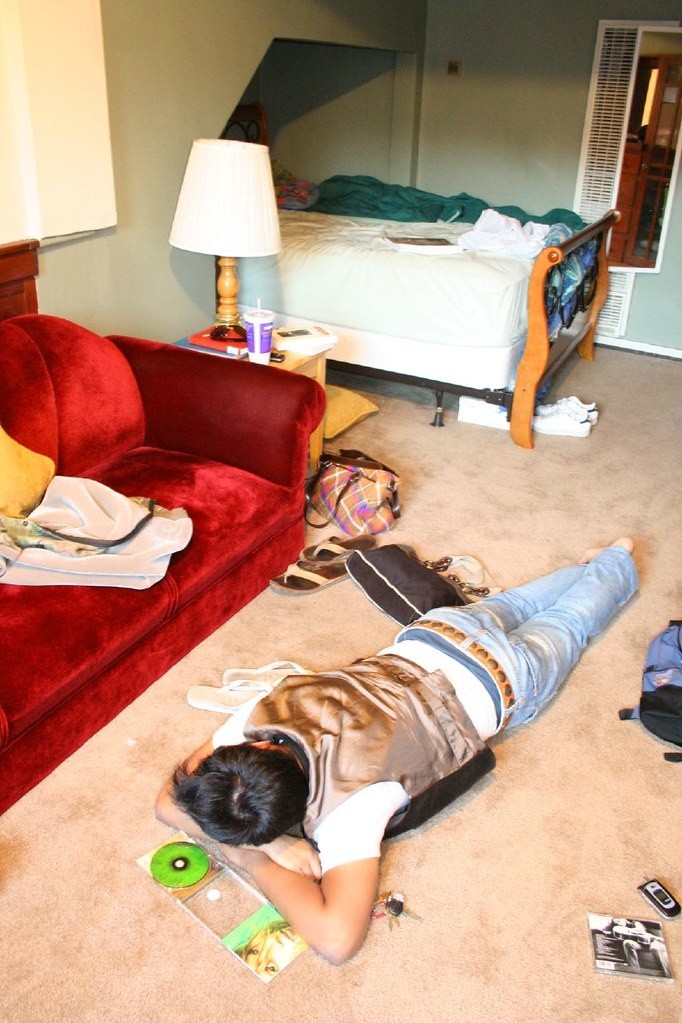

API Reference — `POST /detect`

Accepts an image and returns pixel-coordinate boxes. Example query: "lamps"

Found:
[168,135,281,358]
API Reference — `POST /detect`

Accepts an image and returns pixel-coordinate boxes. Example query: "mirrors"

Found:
[603,24,682,274]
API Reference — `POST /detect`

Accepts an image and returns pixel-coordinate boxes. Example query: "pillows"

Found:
[322,384,379,440]
[0,423,56,518]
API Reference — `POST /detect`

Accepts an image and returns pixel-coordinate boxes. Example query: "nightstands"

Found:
[173,321,334,470]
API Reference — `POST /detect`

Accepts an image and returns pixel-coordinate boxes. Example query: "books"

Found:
[382,238,455,254]
[173,336,247,359]
[188,325,248,355]
[272,326,337,351]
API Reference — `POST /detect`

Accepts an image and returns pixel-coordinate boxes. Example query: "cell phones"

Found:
[637,876,682,920]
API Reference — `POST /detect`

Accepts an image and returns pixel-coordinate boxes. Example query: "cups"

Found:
[242,309,275,366]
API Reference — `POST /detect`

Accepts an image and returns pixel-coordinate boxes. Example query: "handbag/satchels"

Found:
[344,544,490,628]
[304,451,402,538]
[618,620,682,762]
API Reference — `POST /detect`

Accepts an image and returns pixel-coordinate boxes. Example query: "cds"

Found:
[151,842,210,889]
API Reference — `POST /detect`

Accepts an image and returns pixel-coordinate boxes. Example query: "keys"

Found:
[382,893,424,931]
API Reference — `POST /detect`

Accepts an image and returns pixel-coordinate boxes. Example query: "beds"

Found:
[219,190,620,449]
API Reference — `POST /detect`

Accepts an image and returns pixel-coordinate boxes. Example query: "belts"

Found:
[407,621,515,738]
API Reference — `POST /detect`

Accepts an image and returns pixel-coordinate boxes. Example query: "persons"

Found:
[156,536,639,966]
[622,920,672,978]
[234,921,308,983]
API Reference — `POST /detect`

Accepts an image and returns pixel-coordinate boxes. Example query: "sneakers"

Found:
[531,396,599,437]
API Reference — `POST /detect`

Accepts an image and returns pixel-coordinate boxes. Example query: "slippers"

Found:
[269,561,355,596]
[186,660,315,714]
[299,534,382,565]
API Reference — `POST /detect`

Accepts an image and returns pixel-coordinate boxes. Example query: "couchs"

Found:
[0,315,329,818]
